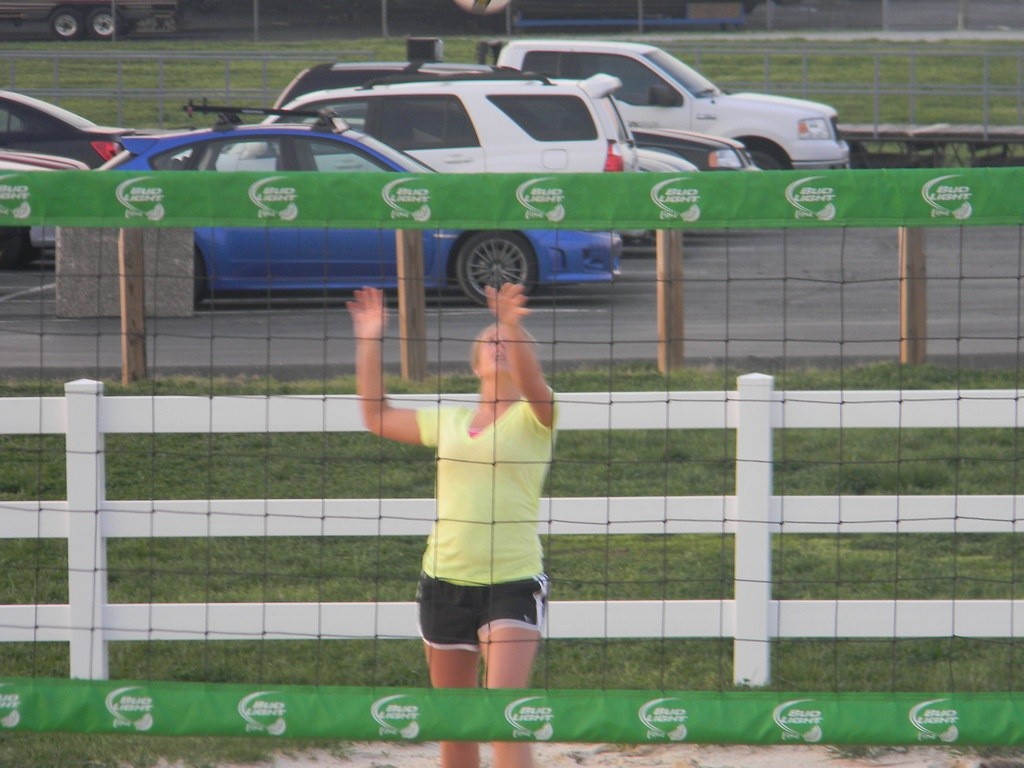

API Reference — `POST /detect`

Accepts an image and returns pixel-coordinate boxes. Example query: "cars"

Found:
[1,86,133,270]
[2,150,94,272]
[617,146,704,250]
[53,109,624,315]
[1,1,183,44]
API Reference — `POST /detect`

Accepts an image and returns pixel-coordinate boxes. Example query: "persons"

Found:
[344,283,559,768]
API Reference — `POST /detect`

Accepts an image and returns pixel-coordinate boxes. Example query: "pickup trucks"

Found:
[489,37,853,193]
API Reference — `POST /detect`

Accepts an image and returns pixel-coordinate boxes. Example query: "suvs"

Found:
[244,73,641,245]
[267,64,769,229]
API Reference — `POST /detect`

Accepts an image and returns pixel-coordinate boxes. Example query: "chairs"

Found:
[368,109,418,148]
